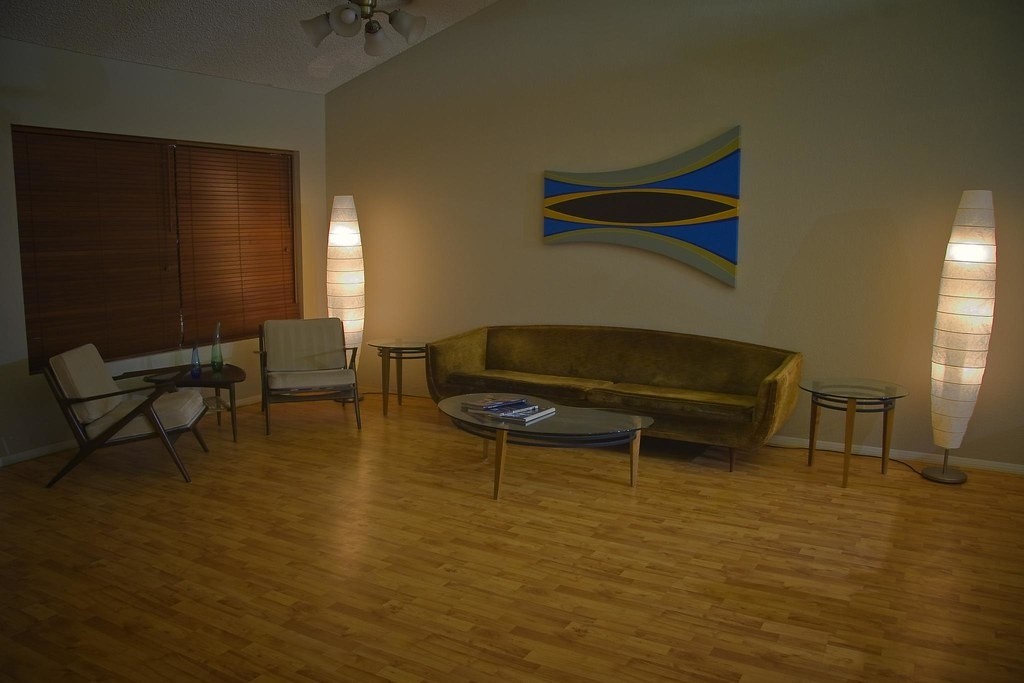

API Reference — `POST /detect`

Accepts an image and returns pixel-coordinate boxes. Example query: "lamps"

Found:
[299,0,427,57]
[326,195,366,403]
[917,190,996,486]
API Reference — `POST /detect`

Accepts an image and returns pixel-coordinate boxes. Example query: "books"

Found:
[461,396,555,423]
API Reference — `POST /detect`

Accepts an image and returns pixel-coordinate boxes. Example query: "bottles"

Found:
[192,344,201,378]
[211,321,223,372]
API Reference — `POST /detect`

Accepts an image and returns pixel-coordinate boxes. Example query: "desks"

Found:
[143,363,247,443]
[796,376,911,489]
[367,337,433,415]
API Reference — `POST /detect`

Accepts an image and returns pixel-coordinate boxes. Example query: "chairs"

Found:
[44,343,209,489]
[258,318,362,435]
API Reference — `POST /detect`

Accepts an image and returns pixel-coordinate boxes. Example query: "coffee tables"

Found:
[437,392,657,502]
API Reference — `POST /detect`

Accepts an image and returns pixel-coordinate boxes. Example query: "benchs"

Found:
[425,324,804,473]
